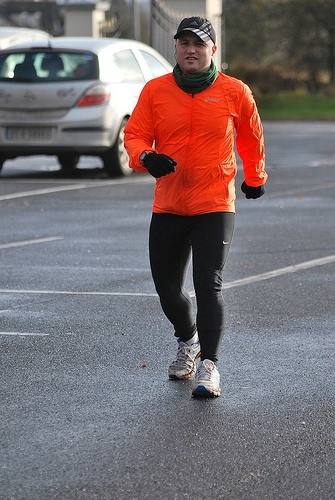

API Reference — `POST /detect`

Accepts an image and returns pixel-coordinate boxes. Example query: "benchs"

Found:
[14,59,94,79]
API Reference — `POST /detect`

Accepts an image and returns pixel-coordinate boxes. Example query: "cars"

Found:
[0,26,175,179]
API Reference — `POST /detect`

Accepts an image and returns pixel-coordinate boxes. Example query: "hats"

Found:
[173,17,216,45]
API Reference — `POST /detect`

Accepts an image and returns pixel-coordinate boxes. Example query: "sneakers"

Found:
[191,358,222,398]
[167,337,204,379]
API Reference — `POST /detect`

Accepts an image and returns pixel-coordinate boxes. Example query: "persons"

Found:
[124,17,268,396]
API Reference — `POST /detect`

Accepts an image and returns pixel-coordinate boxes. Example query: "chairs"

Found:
[41,55,64,77]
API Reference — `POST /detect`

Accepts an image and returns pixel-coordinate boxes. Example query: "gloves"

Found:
[140,150,178,178]
[240,181,266,200]
